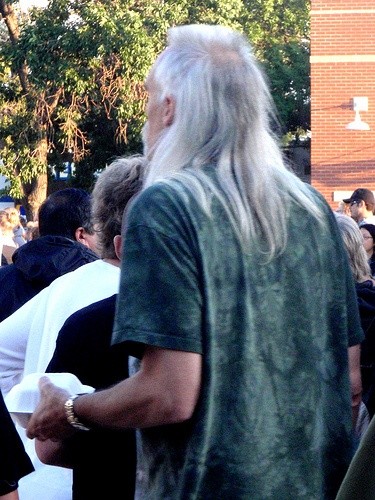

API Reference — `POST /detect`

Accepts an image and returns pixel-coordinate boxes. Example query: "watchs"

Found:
[64,393,92,431]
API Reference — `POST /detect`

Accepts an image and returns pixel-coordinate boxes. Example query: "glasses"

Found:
[350,201,359,206]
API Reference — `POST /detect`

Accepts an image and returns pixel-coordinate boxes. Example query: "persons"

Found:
[334,186,375,452]
[35,190,138,500]
[1,154,151,500]
[1,192,39,267]
[0,186,103,322]
[26,24,362,500]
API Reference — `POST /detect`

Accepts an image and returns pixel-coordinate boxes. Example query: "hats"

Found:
[360,224,375,243]
[343,188,375,206]
